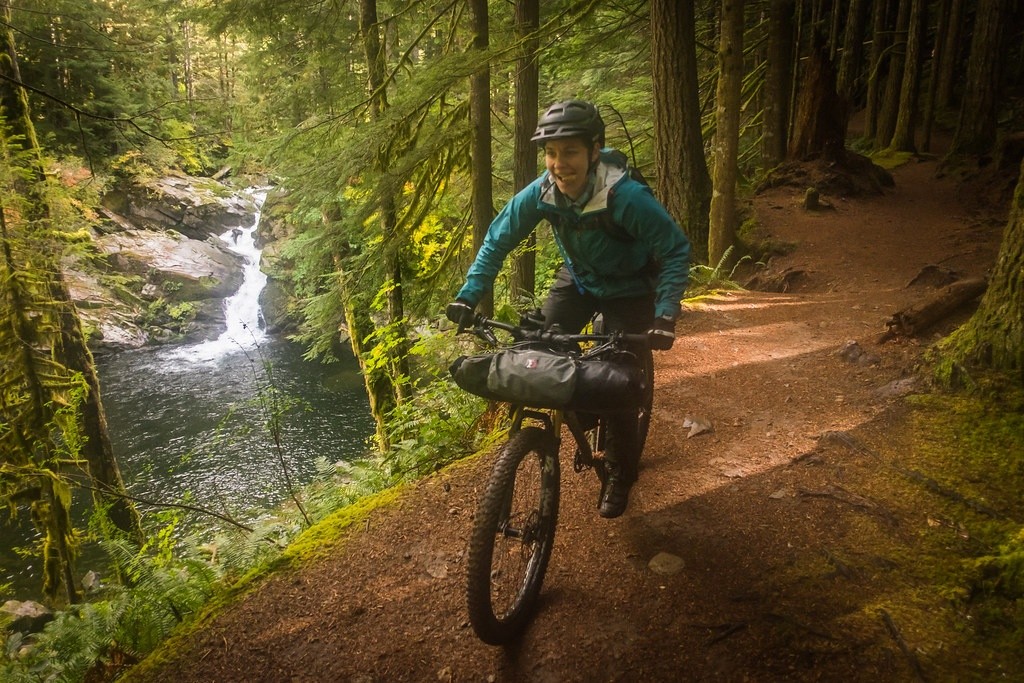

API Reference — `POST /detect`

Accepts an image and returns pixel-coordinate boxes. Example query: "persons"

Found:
[444,99,692,520]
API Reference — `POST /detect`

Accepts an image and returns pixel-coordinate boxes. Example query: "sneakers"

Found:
[573,411,598,431]
[600,477,634,519]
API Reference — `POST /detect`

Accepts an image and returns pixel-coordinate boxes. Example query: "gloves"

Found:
[640,318,675,351]
[446,298,474,334]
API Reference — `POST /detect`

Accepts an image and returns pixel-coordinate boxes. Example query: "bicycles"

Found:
[455,305,653,647]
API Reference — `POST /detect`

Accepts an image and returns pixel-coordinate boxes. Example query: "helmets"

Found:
[530,100,605,148]
